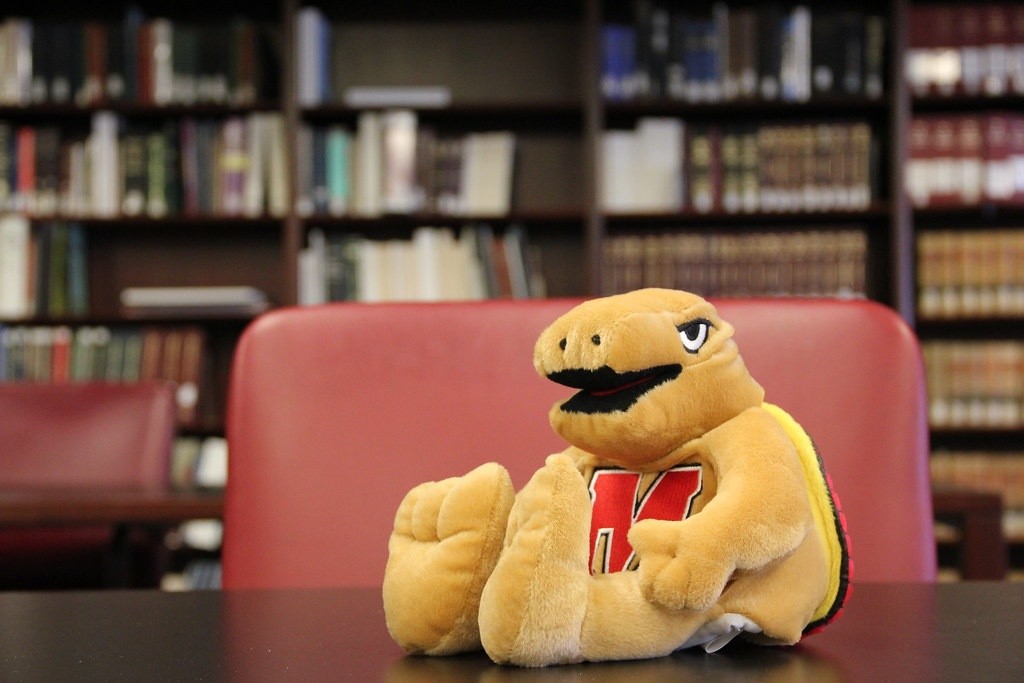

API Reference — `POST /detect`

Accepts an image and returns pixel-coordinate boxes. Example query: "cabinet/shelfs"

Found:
[0,0,1023,579]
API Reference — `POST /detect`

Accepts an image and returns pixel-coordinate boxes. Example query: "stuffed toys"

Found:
[377,285,850,668]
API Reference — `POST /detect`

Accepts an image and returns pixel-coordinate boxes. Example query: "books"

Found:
[296,123,555,306]
[901,1,1023,584]
[595,1,879,303]
[298,10,328,104]
[344,81,453,107]
[0,14,288,495]
